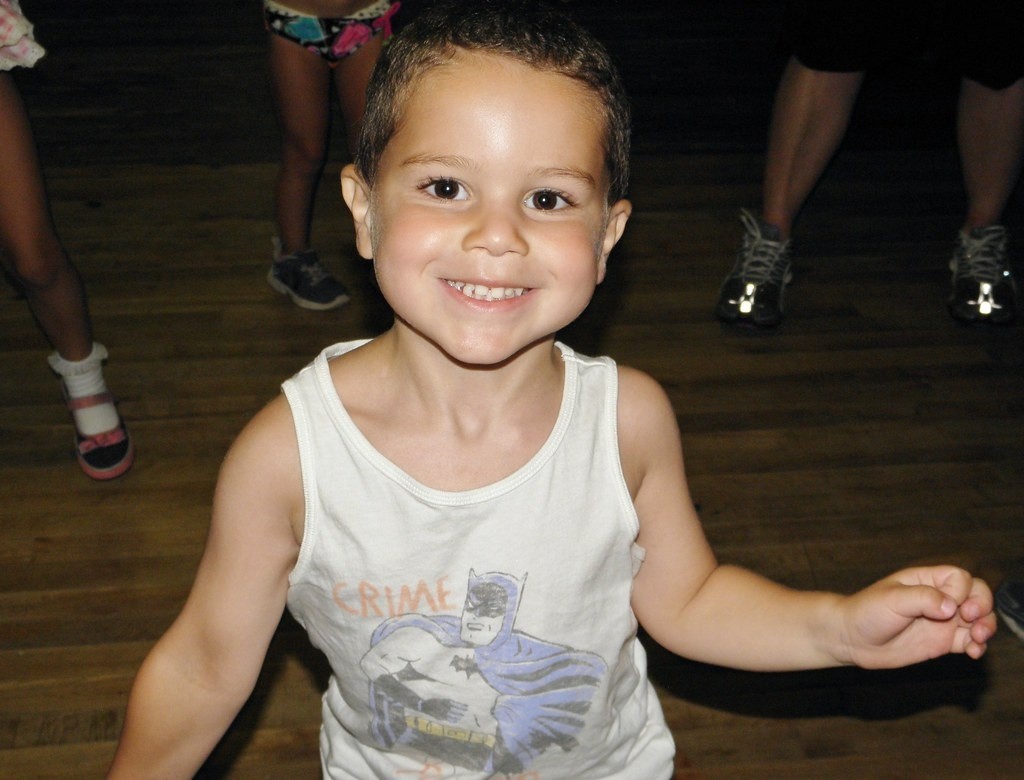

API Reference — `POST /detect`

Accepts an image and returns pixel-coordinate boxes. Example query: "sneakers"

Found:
[945,225,1019,324]
[716,205,795,327]
[266,236,352,310]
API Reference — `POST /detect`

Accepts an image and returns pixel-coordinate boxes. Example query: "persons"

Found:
[262,0,403,311]
[711,1,1024,336]
[108,0,997,780]
[0,0,134,480]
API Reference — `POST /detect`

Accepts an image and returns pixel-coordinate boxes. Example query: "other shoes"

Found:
[59,381,132,480]
